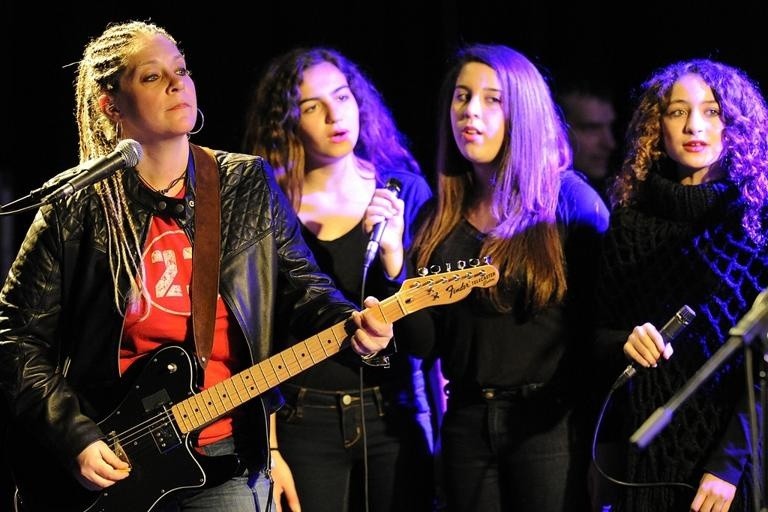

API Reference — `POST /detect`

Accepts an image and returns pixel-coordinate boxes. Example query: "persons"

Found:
[244,46,436,512]
[549,83,623,199]
[1,22,399,512]
[585,57,768,512]
[362,44,611,508]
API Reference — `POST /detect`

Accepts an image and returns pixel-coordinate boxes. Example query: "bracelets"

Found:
[269,443,279,454]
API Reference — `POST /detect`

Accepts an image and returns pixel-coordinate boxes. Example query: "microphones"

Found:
[363,178,405,272]
[44,135,145,205]
[620,302,698,383]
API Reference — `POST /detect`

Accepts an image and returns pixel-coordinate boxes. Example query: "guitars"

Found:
[11,253,499,512]
